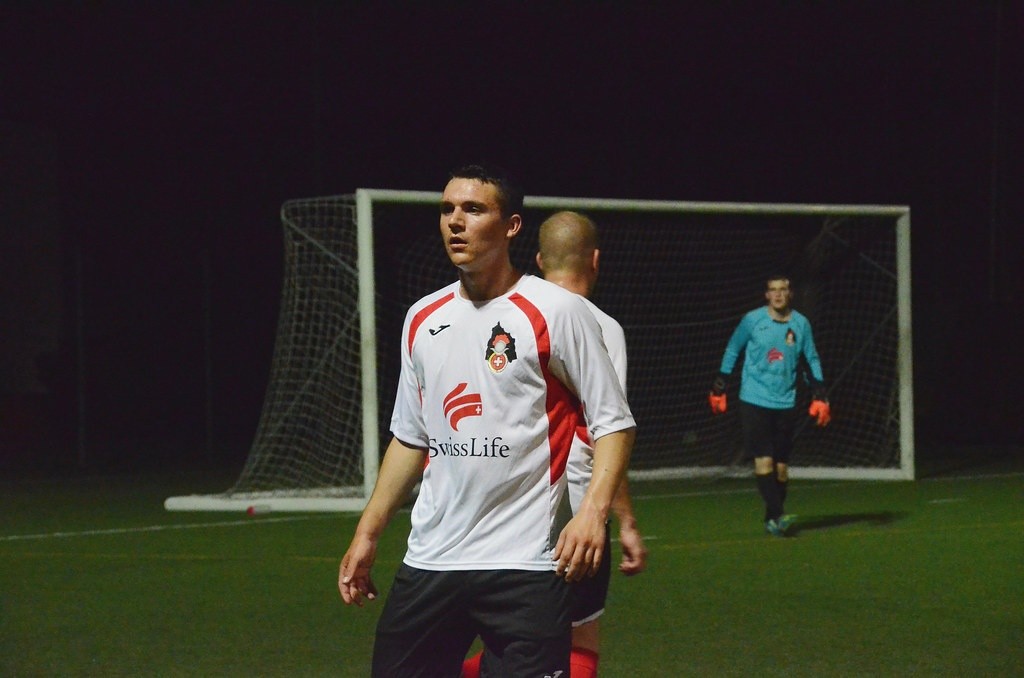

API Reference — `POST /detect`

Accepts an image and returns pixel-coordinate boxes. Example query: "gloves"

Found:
[809,386,830,428]
[708,379,729,413]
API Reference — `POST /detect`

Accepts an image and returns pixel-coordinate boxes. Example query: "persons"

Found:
[337,158,638,678]
[709,274,831,534]
[463,210,647,678]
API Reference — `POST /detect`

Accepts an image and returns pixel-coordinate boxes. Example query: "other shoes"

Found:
[778,512,796,533]
[766,517,778,533]
